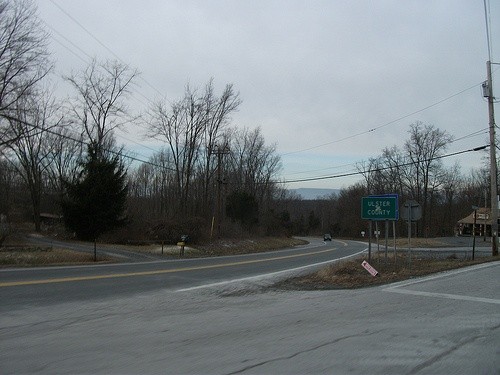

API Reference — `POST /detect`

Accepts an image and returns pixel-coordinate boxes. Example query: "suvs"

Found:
[324,234,332,241]
